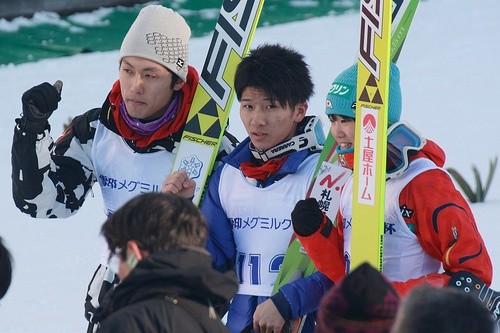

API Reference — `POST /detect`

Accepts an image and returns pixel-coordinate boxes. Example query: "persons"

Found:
[0,235,15,300]
[92,192,239,333]
[291,57,500,333]
[11,4,241,333]
[160,42,335,333]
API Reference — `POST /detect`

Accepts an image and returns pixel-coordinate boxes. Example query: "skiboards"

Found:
[127,0,266,270]
[350,0,392,277]
[271,0,419,333]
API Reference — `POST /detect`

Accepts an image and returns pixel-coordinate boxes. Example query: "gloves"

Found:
[21,79,64,120]
[291,198,326,237]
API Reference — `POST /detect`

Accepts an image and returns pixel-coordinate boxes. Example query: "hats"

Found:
[324,61,401,127]
[120,5,193,83]
[316,261,402,333]
